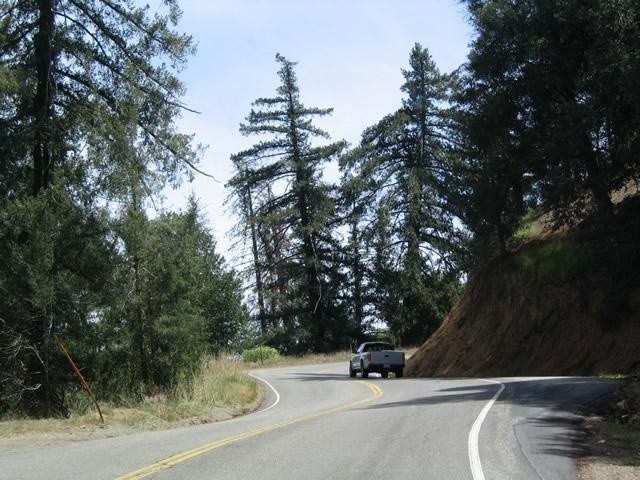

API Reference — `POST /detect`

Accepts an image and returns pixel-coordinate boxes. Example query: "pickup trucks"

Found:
[349,340,406,377]
